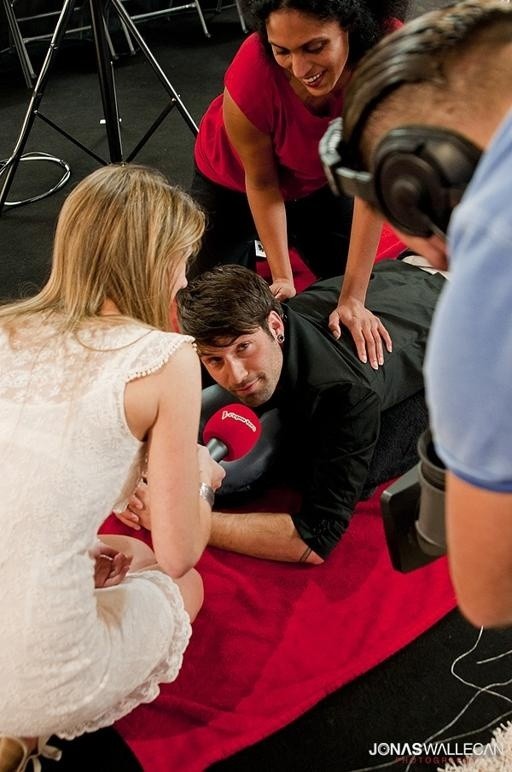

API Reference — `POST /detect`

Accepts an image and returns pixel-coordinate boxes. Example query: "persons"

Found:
[109,247,449,577]
[192,0,394,369]
[327,1,512,630]
[0,161,225,772]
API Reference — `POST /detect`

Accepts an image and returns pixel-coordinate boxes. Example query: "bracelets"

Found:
[197,477,219,507]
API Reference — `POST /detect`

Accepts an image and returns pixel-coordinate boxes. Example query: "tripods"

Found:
[2,0,199,207]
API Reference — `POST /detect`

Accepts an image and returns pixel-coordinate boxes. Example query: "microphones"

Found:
[202,402,262,463]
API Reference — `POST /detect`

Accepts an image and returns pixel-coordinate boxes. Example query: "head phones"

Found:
[317,118,483,238]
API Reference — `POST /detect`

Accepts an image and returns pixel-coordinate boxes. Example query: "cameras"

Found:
[379,427,446,574]
